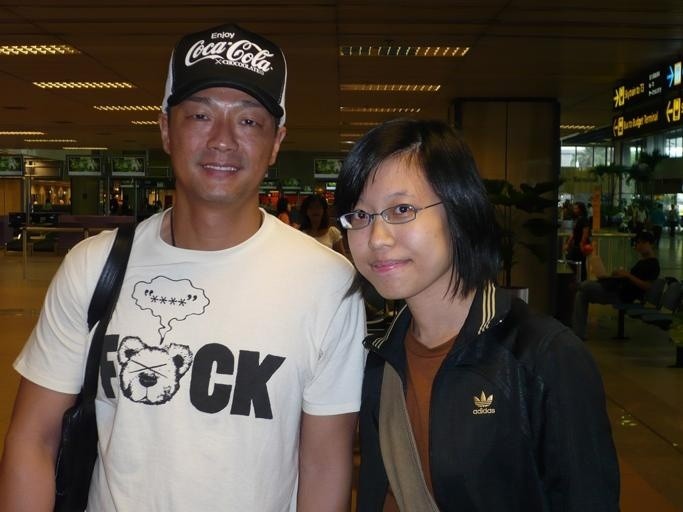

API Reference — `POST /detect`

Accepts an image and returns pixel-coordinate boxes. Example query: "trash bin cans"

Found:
[551,259,582,327]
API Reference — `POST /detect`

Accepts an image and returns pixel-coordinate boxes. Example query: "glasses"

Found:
[337,201,444,230]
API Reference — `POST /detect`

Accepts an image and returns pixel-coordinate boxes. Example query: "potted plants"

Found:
[484,177,568,304]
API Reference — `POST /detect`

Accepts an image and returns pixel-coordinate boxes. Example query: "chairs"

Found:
[610,277,683,340]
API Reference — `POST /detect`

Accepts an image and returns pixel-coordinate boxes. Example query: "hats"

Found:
[161,22,286,129]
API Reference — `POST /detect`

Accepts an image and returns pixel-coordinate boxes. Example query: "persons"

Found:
[296,192,347,258]
[331,115,623,512]
[563,200,595,281]
[274,197,293,226]
[0,21,368,512]
[576,230,660,342]
[559,199,680,251]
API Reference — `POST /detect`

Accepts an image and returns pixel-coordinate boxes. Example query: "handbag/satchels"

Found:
[52,395,99,512]
